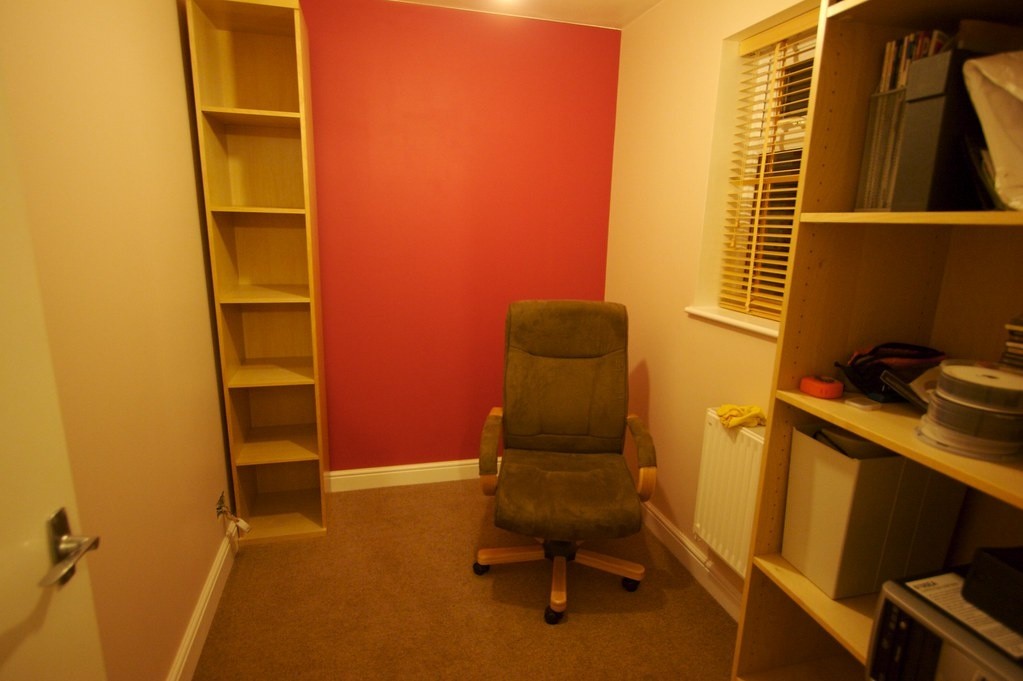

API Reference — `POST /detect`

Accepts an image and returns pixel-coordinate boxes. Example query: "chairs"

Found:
[471,298,658,625]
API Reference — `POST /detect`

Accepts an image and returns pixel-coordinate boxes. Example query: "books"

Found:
[863,30,954,212]
[1002,319,1023,368]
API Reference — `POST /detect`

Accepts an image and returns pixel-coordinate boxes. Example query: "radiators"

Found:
[691,409,764,579]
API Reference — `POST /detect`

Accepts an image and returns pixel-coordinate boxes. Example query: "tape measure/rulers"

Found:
[799,374,844,399]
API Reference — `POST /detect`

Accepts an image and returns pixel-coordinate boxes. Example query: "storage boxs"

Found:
[891,48,1010,213]
[782,424,968,600]
[853,88,911,211]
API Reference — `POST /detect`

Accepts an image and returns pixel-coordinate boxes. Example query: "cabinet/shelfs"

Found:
[187,1,328,548]
[730,0,1023,681]
